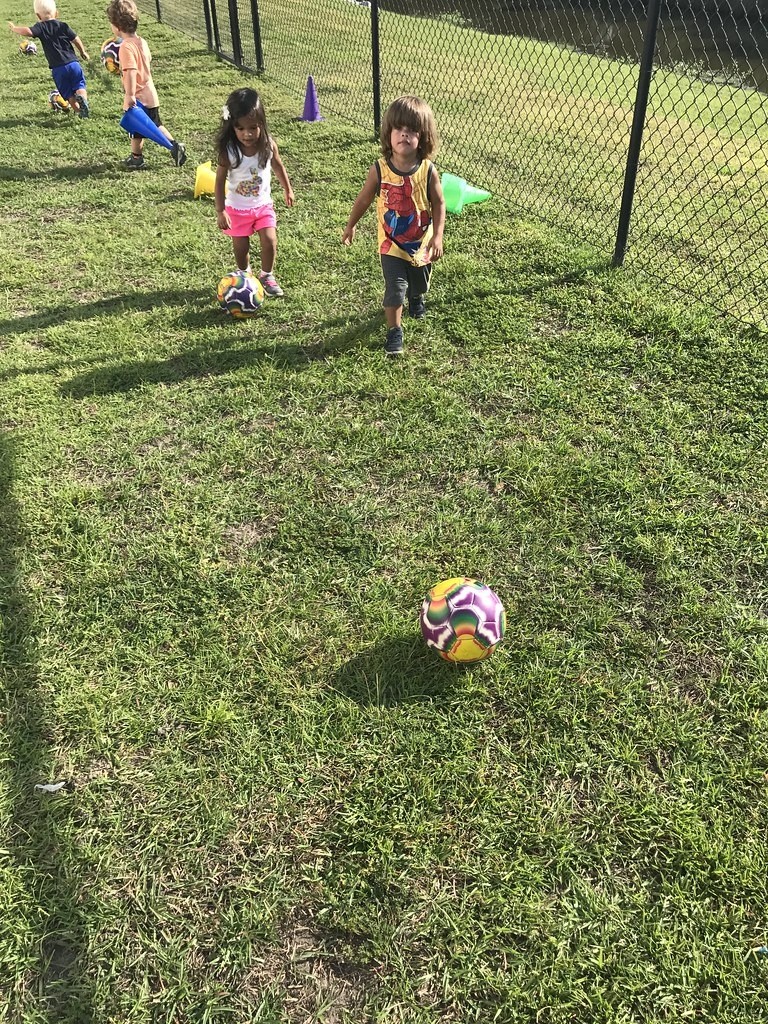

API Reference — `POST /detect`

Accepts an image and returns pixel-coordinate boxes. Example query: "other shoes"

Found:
[76,95,90,118]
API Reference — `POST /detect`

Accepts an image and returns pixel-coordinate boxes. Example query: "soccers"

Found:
[217,269,263,317]
[19,40,35,55]
[422,577,507,662]
[50,91,71,113]
[101,38,123,75]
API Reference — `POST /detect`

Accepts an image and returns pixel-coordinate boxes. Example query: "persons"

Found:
[106,0,187,168]
[342,95,446,354]
[214,87,294,297]
[7,0,89,118]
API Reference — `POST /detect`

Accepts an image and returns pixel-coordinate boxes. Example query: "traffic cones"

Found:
[295,76,325,123]
[119,99,173,150]
[194,161,229,200]
[440,173,493,214]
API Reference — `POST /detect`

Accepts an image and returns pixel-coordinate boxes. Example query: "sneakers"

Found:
[383,326,403,355]
[121,155,147,169]
[406,288,425,318]
[170,142,187,167]
[258,274,284,297]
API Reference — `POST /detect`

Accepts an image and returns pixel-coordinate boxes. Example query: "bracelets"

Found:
[216,206,225,212]
